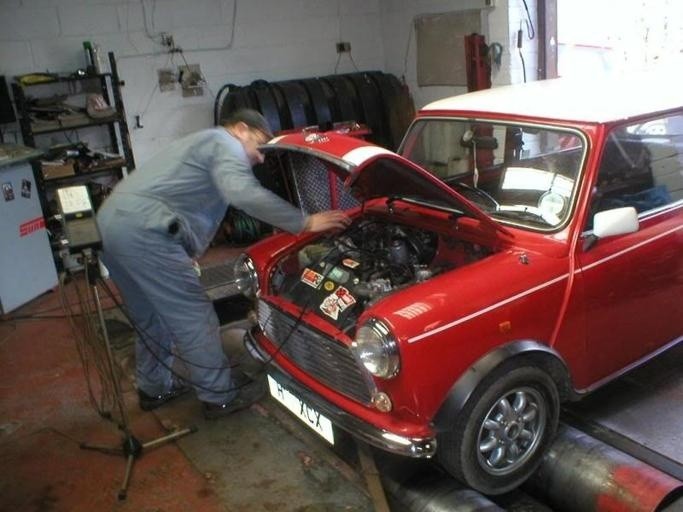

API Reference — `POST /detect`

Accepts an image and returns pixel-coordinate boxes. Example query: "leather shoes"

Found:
[138,379,191,410]
[201,382,266,418]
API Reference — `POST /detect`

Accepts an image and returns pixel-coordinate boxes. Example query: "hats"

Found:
[233,108,274,139]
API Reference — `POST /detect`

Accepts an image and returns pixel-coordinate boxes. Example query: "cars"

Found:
[234,72,683,497]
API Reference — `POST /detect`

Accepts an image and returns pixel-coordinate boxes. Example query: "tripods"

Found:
[79,241,201,501]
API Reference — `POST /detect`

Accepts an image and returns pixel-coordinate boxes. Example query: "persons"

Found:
[94,107,353,421]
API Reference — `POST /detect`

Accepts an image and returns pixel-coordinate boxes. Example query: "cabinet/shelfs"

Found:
[12,41,134,277]
